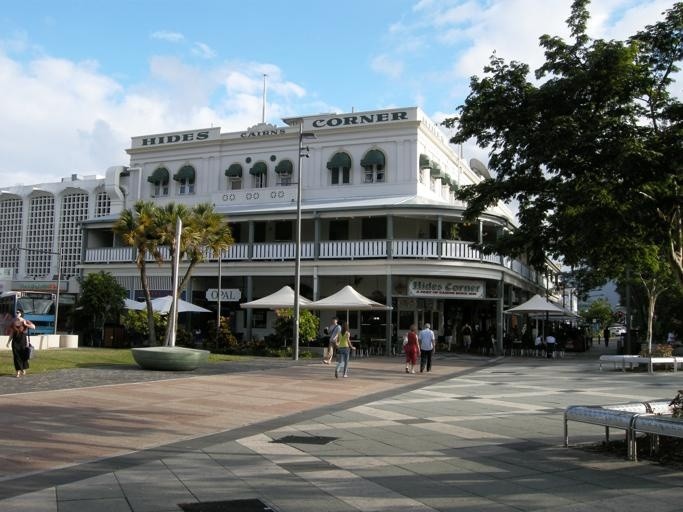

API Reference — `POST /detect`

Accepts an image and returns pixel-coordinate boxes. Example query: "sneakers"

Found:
[405,367,416,373]
[335,371,337,378]
[344,375,349,378]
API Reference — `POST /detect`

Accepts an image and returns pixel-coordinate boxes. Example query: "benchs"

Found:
[599,355,683,376]
[563,400,683,463]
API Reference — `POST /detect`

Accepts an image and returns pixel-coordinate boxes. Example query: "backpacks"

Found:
[463,325,470,335]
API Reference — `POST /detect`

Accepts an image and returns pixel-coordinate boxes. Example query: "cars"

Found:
[608,327,625,337]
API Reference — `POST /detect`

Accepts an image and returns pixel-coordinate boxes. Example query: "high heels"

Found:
[16,370,26,378]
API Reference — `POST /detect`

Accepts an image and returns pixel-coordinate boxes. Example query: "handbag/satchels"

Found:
[25,344,34,360]
[321,336,330,346]
[403,333,408,346]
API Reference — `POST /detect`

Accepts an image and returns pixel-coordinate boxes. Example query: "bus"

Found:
[0,290,56,336]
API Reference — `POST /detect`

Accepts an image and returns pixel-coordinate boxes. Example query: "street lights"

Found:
[292,121,318,361]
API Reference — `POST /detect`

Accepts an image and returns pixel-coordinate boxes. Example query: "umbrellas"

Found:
[239,285,316,348]
[123,297,140,307]
[503,293,584,344]
[304,283,394,332]
[129,294,213,316]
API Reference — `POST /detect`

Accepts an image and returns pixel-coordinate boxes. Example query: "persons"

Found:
[5,306,37,378]
[321,317,344,364]
[402,323,421,374]
[443,319,612,359]
[320,326,330,361]
[333,323,358,378]
[417,321,437,373]
[667,327,678,345]
